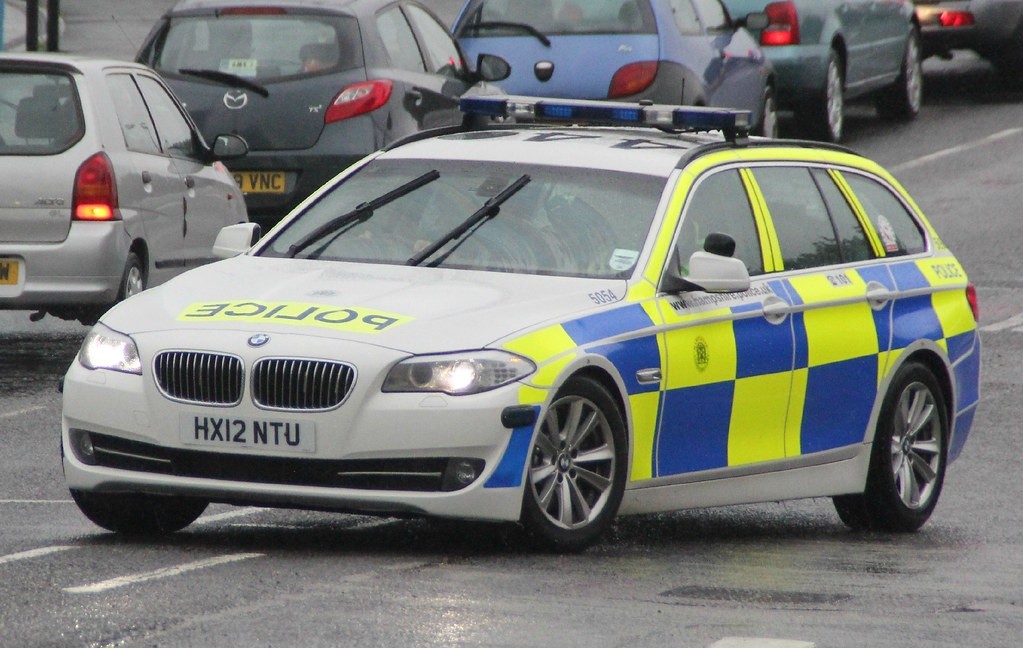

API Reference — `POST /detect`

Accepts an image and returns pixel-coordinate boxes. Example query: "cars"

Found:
[133,0,514,233]
[444,0,784,141]
[0,46,251,327]
[913,1,1023,82]
[728,1,926,146]
[60,97,983,555]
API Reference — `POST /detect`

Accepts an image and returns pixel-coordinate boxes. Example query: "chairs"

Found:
[14,97,60,147]
